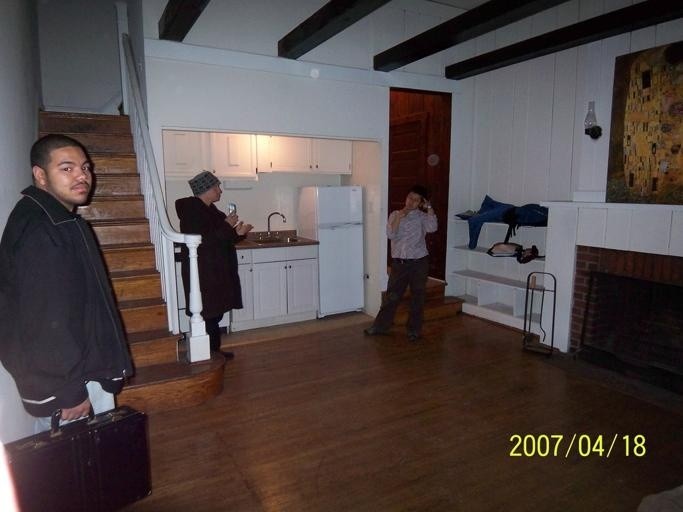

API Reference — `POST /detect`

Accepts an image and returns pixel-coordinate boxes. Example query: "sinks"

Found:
[248,238,307,244]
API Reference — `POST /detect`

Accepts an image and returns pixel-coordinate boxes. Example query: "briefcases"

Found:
[4,404,153,512]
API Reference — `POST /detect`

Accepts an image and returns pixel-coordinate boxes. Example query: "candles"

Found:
[531,274,535,290]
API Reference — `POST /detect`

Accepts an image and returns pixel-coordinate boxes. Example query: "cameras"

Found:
[229,204,236,214]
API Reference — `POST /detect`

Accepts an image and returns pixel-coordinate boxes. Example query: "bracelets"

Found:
[426,205,432,210]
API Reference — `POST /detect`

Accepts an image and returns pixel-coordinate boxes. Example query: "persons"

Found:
[172,170,255,359]
[361,184,438,338]
[0,134,138,435]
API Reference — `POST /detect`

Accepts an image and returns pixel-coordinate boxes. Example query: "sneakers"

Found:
[407,328,416,341]
[220,350,235,361]
[363,326,384,334]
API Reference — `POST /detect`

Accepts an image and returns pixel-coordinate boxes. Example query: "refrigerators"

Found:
[296,185,365,319]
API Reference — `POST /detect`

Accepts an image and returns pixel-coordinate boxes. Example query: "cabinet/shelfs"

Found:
[449,217,548,337]
[162,129,353,181]
[229,247,255,333]
[252,242,320,330]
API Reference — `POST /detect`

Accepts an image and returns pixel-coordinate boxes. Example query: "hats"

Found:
[187,171,221,197]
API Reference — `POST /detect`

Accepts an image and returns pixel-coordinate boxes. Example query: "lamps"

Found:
[584,101,602,140]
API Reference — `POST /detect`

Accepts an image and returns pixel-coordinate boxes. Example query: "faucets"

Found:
[267,211,286,238]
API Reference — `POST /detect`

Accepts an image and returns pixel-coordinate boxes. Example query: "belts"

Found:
[391,256,429,264]
